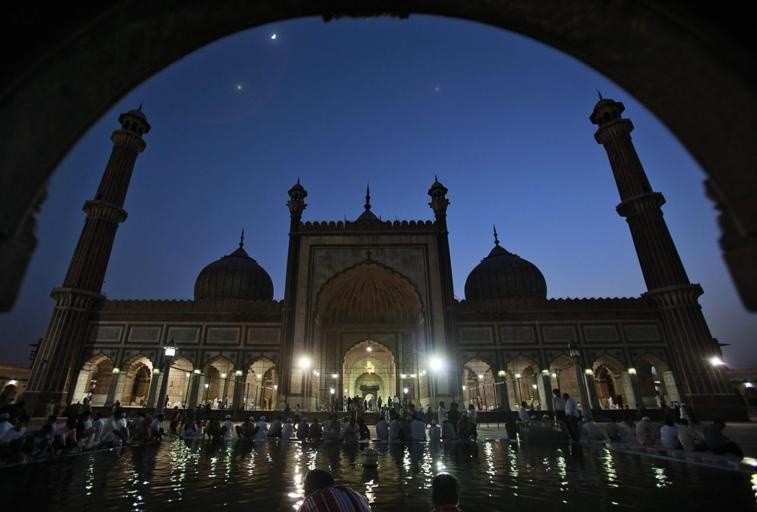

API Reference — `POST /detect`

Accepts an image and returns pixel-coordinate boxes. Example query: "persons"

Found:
[428,473,464,511]
[295,468,372,511]
[514,388,592,441]
[165,395,500,441]
[606,394,744,457]
[1,385,165,471]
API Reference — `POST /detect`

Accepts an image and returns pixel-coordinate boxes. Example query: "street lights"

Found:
[474,369,526,431]
[566,340,593,421]
[154,337,179,419]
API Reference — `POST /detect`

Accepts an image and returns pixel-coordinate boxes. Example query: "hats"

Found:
[528,414,550,419]
[223,413,309,423]
